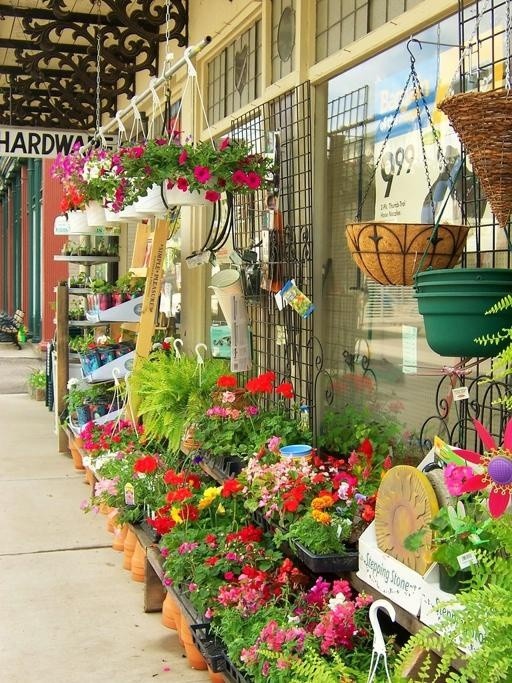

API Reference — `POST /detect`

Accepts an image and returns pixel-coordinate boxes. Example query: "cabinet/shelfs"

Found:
[356,444,512,656]
[51,217,170,440]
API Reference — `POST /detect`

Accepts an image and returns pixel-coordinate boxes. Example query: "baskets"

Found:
[346,222,468,286]
[436,90,511,228]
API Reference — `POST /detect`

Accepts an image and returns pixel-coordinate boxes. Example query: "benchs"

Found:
[0,308,25,349]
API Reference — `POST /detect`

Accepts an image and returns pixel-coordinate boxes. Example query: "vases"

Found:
[346,222,512,356]
[54,192,139,236]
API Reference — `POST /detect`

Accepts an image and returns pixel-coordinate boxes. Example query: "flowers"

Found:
[79,370,428,682]
[49,130,282,213]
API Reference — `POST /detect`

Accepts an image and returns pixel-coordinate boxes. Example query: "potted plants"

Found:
[26,367,46,400]
[127,348,237,453]
